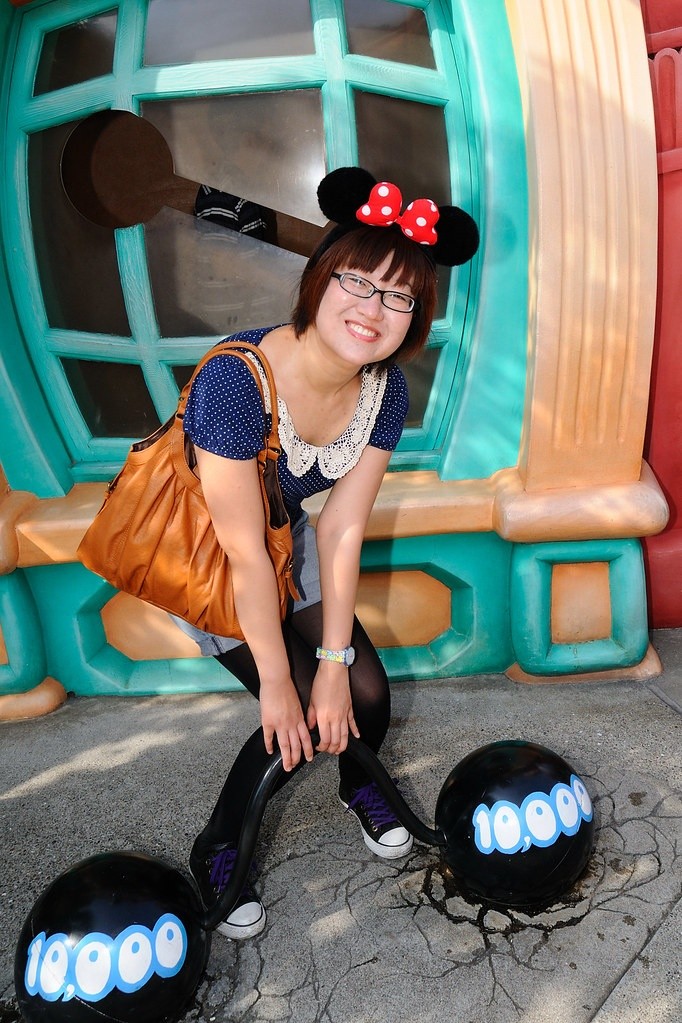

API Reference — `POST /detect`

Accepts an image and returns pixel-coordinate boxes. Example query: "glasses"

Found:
[331,269,419,315]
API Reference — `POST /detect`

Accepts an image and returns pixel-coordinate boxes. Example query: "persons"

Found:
[77,164,479,940]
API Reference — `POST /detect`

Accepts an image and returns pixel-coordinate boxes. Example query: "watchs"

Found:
[315,647,355,667]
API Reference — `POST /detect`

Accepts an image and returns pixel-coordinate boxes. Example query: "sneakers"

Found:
[188,836,266,938]
[337,777,415,859]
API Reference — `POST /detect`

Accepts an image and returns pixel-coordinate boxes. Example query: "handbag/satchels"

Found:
[75,341,300,643]
